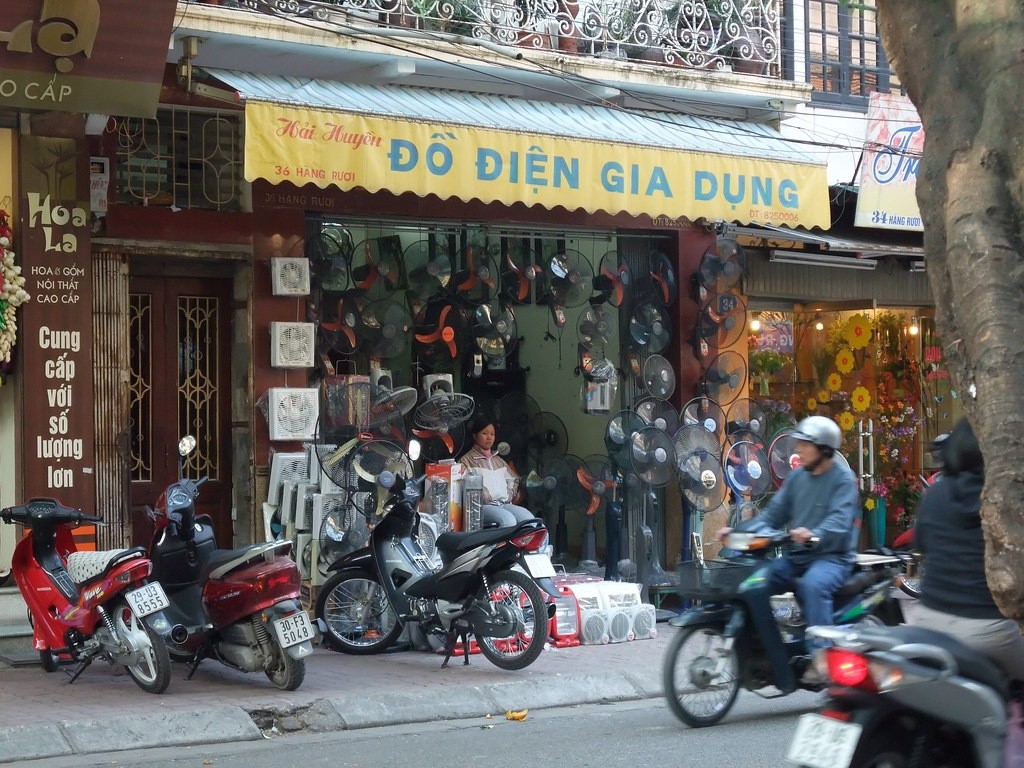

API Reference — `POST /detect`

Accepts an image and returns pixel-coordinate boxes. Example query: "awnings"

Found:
[198,66,830,230]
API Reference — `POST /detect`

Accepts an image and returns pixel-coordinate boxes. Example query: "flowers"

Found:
[747,308,949,529]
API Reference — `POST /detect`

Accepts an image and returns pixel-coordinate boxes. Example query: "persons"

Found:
[717,416,858,684]
[912,418,1024,683]
[458,417,536,547]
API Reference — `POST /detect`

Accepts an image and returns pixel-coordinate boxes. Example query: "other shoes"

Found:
[802,665,821,684]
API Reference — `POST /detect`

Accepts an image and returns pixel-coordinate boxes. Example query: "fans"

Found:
[263,223,802,657]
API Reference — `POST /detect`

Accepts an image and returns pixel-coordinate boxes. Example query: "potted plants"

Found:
[581,0,766,74]
[514,0,549,48]
[313,0,348,23]
[348,0,386,28]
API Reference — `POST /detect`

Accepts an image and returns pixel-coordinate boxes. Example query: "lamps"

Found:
[192,81,246,106]
[910,261,927,273]
[770,249,878,271]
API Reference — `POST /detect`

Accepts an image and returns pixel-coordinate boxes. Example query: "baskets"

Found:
[676,558,754,603]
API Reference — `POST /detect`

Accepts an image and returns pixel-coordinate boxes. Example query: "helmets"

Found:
[791,416,842,450]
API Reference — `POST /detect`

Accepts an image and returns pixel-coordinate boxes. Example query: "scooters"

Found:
[0,496,192,694]
[140,434,315,692]
[782,613,1024,768]
[657,516,909,727]
[312,469,563,674]
